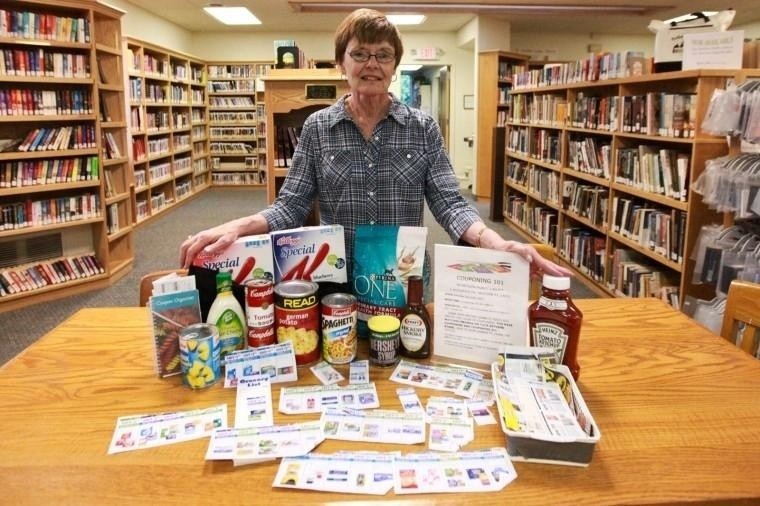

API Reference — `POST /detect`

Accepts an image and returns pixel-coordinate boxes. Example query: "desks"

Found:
[1,295,760,506]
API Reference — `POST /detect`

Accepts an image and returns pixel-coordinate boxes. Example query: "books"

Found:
[207,64,271,184]
[127,48,208,218]
[497,51,698,309]
[0,255,104,298]
[274,126,300,168]
[1,9,121,237]
[272,38,316,70]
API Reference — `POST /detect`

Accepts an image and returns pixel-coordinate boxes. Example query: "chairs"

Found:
[139,270,184,304]
[505,244,552,303]
[719,279,760,358]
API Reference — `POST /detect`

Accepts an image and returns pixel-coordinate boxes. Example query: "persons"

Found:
[176,8,577,287]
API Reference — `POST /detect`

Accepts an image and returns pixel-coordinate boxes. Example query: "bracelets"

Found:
[475,227,485,249]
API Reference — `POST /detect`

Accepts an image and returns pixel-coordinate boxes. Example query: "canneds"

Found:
[321,294,358,365]
[275,281,320,366]
[178,323,221,390]
[245,279,274,327]
[368,315,400,367]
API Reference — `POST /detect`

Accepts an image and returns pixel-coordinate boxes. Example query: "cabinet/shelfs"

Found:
[1,0,135,314]
[123,38,208,231]
[203,60,306,185]
[474,50,527,200]
[489,53,760,315]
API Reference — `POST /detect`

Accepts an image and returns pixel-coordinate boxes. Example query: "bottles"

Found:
[529,272,583,383]
[399,276,431,359]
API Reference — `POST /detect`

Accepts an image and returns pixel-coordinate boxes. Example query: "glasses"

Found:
[345,45,396,64]
